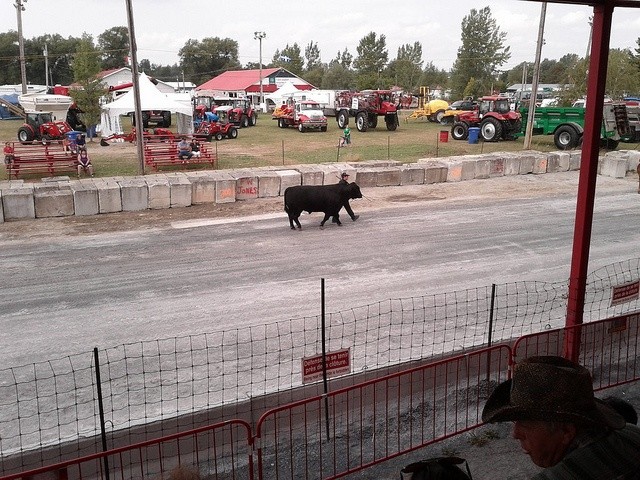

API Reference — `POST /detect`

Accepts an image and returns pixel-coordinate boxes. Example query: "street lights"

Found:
[253,32,266,114]
[13,0,27,94]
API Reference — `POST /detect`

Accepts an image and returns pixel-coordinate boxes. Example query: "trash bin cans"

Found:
[468,127,481,144]
[67,131,82,144]
[86,124,96,137]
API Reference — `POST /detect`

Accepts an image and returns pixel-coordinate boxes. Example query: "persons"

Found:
[599,396,637,425]
[177,137,192,163]
[75,134,87,153]
[343,124,351,144]
[189,138,201,160]
[481,354,640,480]
[399,457,473,480]
[62,136,72,152]
[332,173,360,223]
[3,142,15,168]
[77,150,95,178]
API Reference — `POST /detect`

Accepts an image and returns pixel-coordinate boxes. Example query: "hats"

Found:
[482,356,625,430]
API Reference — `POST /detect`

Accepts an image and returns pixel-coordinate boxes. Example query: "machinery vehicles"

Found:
[197,122,237,140]
[333,88,399,131]
[130,110,172,128]
[451,95,627,150]
[101,127,175,146]
[404,86,462,123]
[18,110,74,146]
[227,108,256,127]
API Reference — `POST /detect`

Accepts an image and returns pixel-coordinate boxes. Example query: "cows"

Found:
[284,182,362,230]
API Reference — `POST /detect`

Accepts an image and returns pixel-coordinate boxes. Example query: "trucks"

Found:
[272,96,328,132]
